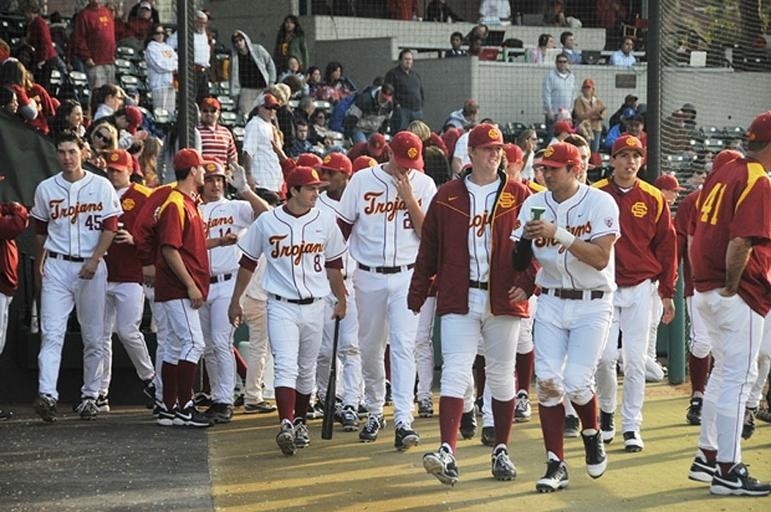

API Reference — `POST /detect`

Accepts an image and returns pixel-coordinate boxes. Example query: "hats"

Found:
[365,132,386,159]
[581,79,595,89]
[551,121,578,135]
[625,94,638,104]
[199,97,222,111]
[256,93,282,109]
[314,151,354,178]
[711,148,745,175]
[743,109,771,143]
[611,134,647,158]
[443,128,460,143]
[652,174,689,192]
[100,147,135,172]
[467,122,506,151]
[387,129,425,172]
[139,1,152,10]
[295,152,323,170]
[124,104,144,138]
[231,32,244,39]
[540,142,583,173]
[463,98,480,110]
[380,82,395,102]
[171,147,216,172]
[280,157,297,182]
[556,106,573,122]
[131,156,146,178]
[501,143,525,165]
[352,154,380,174]
[286,165,330,187]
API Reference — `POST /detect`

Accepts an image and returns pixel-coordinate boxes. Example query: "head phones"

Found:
[561,32,572,44]
[539,34,553,45]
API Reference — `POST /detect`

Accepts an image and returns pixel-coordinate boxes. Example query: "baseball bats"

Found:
[321,302,340,440]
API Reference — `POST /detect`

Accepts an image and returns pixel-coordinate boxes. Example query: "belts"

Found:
[275,294,322,306]
[48,249,85,264]
[209,273,233,285]
[342,275,347,281]
[469,280,488,291]
[542,287,604,301]
[359,262,416,275]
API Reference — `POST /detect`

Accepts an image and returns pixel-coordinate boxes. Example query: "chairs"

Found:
[489,119,746,217]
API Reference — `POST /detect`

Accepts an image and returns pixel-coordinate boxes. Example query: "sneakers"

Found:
[422,440,460,488]
[490,443,517,482]
[474,394,485,417]
[709,462,771,498]
[0,409,9,422]
[243,400,277,415]
[314,401,325,418]
[382,379,394,407]
[172,400,215,427]
[192,393,212,406]
[189,402,234,426]
[741,406,758,441]
[306,403,317,421]
[151,400,162,417]
[755,407,771,423]
[73,395,110,415]
[685,396,703,425]
[358,412,388,444]
[578,427,609,481]
[340,405,361,432]
[481,425,496,448]
[562,414,583,438]
[623,430,645,454]
[534,450,569,494]
[333,397,343,423]
[395,423,420,453]
[598,409,616,445]
[687,448,717,484]
[79,397,99,421]
[417,396,433,419]
[357,405,369,420]
[33,393,58,423]
[156,401,178,427]
[459,408,479,441]
[292,416,311,448]
[142,378,164,408]
[512,389,534,422]
[275,418,297,457]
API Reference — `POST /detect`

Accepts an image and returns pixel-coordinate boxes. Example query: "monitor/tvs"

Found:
[480,46,498,61]
[689,51,707,67]
[485,31,505,46]
[544,48,563,64]
[582,49,600,64]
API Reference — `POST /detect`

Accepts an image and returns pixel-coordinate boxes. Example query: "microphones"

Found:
[553,47,556,49]
[575,44,578,46]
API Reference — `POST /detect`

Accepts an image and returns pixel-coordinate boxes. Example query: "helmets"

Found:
[197,160,228,181]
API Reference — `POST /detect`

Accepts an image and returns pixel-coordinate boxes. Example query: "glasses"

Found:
[316,115,326,120]
[203,109,216,113]
[233,39,244,43]
[153,31,165,35]
[263,105,277,110]
[556,60,568,63]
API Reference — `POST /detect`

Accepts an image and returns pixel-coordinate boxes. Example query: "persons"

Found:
[0,0,771,496]
